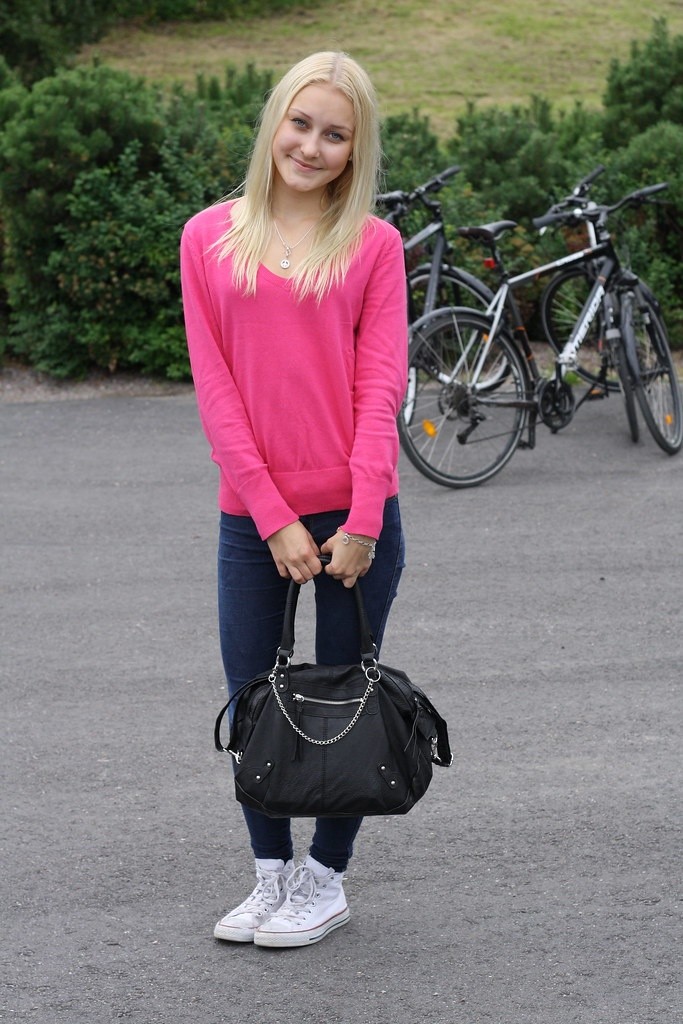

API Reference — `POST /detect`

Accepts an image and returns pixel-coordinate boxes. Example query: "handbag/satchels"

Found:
[213,554,453,818]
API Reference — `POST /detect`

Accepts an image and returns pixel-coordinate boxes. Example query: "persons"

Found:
[179,53,409,947]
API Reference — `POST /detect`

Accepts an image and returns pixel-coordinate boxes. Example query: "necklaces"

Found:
[272,221,320,269]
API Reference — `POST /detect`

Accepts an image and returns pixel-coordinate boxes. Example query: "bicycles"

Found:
[397,165,683,488]
[375,165,514,426]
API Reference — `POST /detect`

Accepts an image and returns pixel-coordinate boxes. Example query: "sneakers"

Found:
[214,857,296,942]
[254,856,352,948]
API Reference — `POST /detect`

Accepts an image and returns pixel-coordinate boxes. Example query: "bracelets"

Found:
[336,527,377,560]
